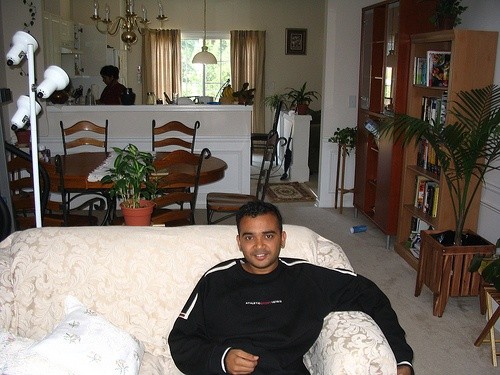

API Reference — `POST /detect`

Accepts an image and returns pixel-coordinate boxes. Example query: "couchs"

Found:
[0,224,399,375]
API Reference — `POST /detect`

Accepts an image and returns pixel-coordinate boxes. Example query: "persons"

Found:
[168,200,415,375]
[96,65,126,105]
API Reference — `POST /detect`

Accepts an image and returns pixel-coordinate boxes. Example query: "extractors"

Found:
[61,47,85,55]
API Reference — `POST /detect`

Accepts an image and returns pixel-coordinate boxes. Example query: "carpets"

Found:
[267,182,316,203]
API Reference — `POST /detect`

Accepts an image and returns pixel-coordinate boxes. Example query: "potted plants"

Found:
[262,81,321,115]
[328,127,360,157]
[467,239,500,314]
[100,143,169,226]
[430,0,469,30]
[360,84,500,317]
[232,83,256,106]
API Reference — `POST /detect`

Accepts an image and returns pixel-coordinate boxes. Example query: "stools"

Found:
[474,287,500,367]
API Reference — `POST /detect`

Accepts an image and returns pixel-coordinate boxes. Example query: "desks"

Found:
[31,151,228,188]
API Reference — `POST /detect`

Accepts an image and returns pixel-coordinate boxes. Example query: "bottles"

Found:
[84,94,95,105]
[350,224,368,234]
[146,92,155,105]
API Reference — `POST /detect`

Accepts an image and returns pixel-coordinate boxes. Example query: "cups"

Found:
[172,92,178,105]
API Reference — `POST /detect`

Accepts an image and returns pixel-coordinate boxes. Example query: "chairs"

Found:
[8,101,282,235]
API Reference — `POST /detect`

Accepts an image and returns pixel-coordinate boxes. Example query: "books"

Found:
[409,216,430,242]
[416,138,443,176]
[414,176,439,217]
[420,95,448,130]
[413,50,451,88]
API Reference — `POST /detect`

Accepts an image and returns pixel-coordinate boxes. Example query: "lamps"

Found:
[4,31,70,229]
[90,0,169,50]
[192,0,218,64]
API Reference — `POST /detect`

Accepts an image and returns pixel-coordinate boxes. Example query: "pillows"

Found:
[4,309,145,375]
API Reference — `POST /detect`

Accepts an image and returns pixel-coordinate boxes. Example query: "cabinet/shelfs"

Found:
[43,11,91,78]
[353,0,498,271]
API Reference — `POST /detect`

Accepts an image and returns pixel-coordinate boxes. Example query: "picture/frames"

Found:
[285,28,307,55]
